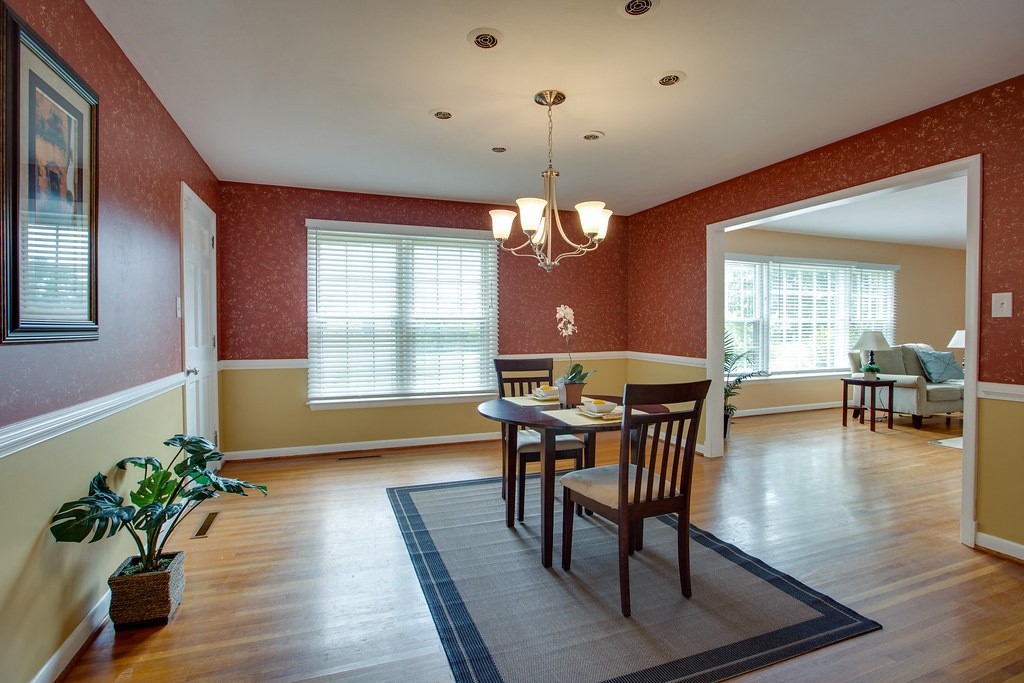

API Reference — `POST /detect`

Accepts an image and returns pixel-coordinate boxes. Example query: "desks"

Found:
[480,394,671,568]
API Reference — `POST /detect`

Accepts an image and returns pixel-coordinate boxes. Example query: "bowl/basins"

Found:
[583,400,617,413]
[535,386,559,397]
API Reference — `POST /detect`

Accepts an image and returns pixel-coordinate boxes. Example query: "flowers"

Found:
[556,304,598,383]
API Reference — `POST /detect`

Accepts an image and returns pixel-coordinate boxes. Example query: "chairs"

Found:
[558,379,712,618]
[492,358,586,524]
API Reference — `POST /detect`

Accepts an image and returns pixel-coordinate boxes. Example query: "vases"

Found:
[558,383,587,408]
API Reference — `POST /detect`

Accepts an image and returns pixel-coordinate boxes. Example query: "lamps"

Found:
[488,89,613,273]
[947,330,965,373]
[852,329,893,380]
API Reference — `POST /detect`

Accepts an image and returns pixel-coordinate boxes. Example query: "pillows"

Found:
[859,346,906,375]
[918,350,965,382]
[901,344,935,380]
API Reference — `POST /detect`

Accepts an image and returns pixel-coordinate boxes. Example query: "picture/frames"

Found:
[0,0,101,348]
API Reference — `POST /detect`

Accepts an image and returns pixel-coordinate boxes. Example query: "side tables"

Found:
[840,378,897,431]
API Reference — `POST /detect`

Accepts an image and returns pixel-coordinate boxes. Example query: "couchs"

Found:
[848,350,964,430]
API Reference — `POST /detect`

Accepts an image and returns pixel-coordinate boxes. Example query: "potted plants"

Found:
[859,363,881,381]
[724,328,771,436]
[52,434,268,629]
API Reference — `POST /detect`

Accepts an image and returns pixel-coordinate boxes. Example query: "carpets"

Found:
[926,436,963,449]
[386,469,884,683]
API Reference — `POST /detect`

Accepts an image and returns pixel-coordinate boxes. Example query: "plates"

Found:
[578,410,622,418]
[524,394,559,401]
